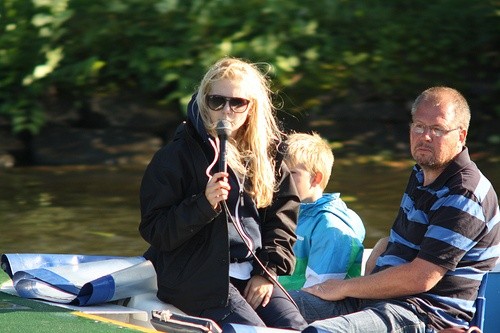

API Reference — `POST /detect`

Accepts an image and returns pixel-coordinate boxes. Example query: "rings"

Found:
[316,286,321,290]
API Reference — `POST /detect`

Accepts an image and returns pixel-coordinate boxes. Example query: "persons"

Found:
[288,86,500,333]
[277,133,365,291]
[138,57,308,331]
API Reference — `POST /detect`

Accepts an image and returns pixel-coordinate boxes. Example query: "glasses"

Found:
[206,94,251,112]
[408,121,460,137]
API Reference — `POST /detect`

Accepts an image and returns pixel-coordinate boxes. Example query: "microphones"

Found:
[216,120,232,185]
